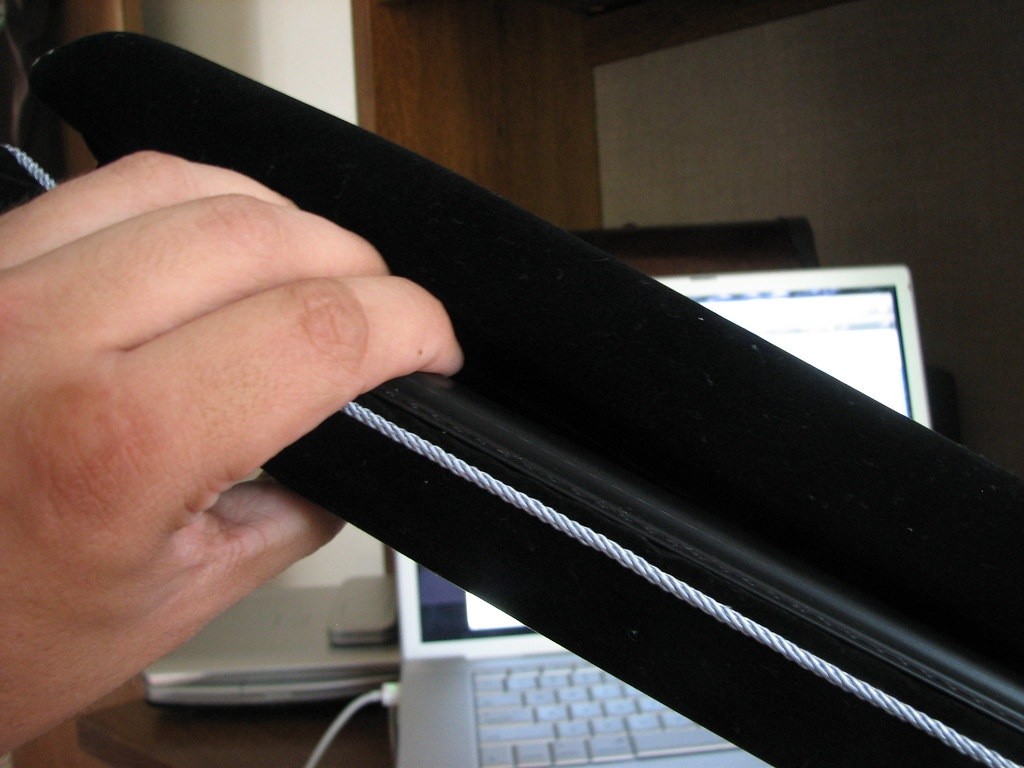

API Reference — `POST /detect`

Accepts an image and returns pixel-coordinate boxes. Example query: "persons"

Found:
[0,150,467,753]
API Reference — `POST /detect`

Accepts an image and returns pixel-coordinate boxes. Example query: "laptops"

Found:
[138,521,387,712]
[380,264,938,768]
